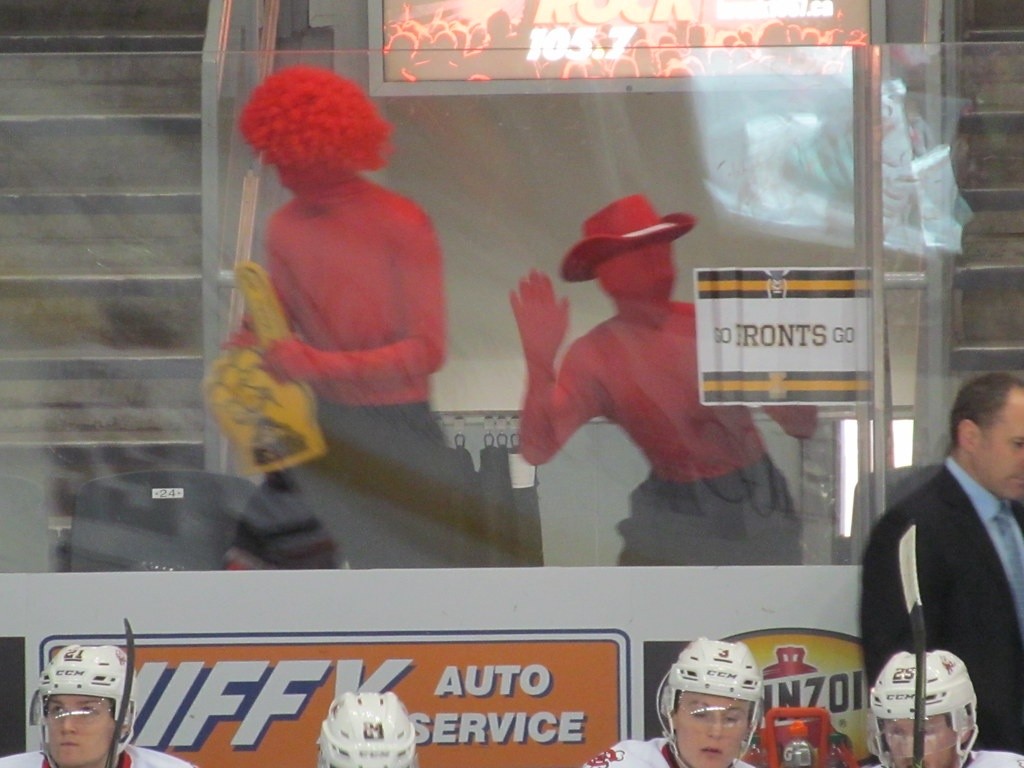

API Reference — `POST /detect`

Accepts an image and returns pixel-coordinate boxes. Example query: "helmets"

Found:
[38,643,137,731]
[656,636,765,726]
[865,649,979,768]
[314,690,420,768]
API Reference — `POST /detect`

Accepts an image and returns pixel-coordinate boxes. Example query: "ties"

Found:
[993,501,1024,651]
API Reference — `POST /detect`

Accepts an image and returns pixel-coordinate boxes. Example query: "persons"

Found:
[0,645,198,768]
[508,193,815,566]
[861,650,1024,768]
[219,65,447,569]
[316,691,417,768]
[860,371,1024,756]
[582,637,765,768]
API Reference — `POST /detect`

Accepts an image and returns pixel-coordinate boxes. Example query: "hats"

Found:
[562,194,697,282]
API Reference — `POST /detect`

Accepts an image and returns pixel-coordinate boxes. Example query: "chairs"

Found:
[0,472,265,574]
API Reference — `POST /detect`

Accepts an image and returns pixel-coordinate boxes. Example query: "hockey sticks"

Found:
[106,618,134,768]
[896,525,928,768]
